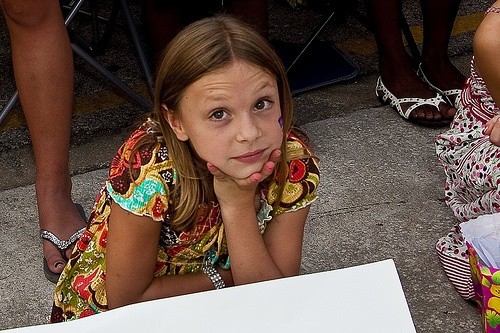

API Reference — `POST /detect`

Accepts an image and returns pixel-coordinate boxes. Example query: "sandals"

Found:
[375,59,471,129]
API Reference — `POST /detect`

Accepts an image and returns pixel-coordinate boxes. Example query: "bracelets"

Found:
[201,265,226,291]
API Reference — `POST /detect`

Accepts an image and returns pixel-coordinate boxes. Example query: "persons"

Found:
[434,0,500,333]
[49,15,320,325]
[366,0,469,127]
[144,0,268,78]
[0,0,90,282]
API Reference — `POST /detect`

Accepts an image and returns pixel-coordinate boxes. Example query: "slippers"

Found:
[43,200,90,285]
